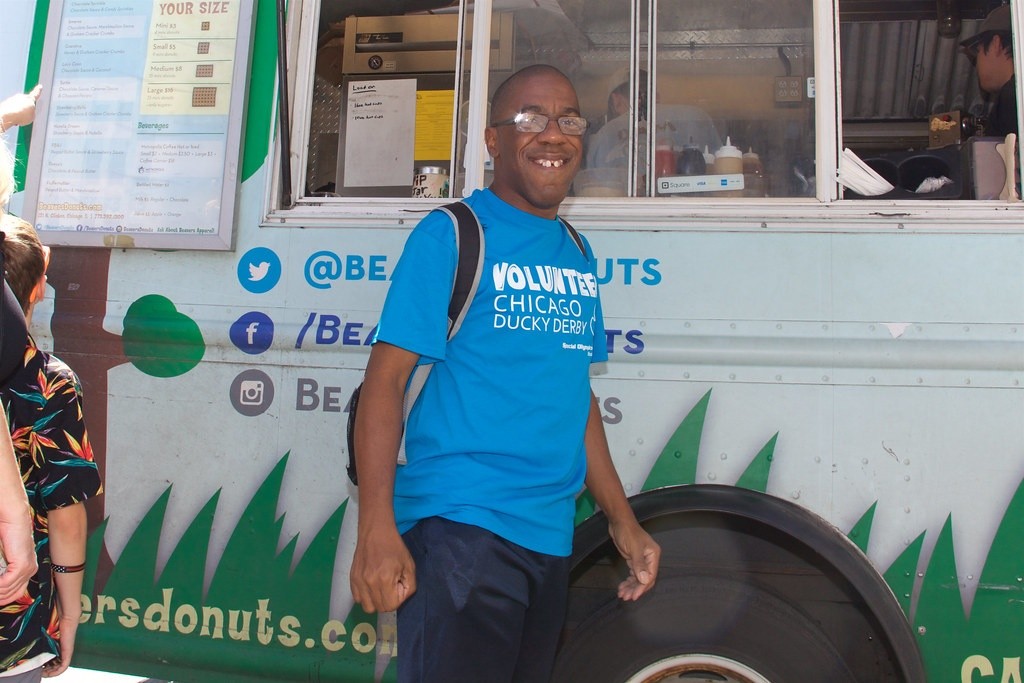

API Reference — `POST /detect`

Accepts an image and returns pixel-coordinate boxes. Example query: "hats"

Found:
[959,5,1011,47]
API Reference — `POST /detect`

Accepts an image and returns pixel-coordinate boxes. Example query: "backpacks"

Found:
[345,201,588,487]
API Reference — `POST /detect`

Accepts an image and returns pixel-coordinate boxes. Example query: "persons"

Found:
[350,65,660,683]
[586,69,724,188]
[960,4,1018,138]
[0,85,105,683]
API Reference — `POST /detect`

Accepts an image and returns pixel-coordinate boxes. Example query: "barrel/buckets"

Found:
[572,168,628,197]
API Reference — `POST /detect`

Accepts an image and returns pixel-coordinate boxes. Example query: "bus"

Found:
[0,0,1024,683]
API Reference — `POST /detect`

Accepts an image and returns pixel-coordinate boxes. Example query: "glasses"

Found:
[493,111,589,137]
[966,39,983,58]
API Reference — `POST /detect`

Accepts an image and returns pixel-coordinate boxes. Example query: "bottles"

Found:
[656,137,764,198]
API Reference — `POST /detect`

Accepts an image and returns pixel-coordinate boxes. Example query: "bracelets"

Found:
[52,562,86,573]
